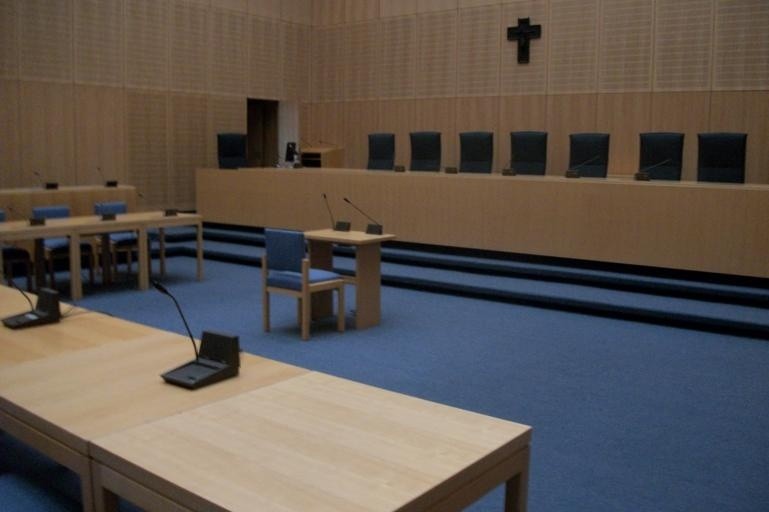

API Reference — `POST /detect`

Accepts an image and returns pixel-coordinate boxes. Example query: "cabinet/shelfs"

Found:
[300,145,344,168]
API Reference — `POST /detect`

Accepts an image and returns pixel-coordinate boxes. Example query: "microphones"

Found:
[565,154,601,178]
[636,158,671,181]
[151,279,240,390]
[7,205,45,227]
[35,171,58,190]
[343,197,382,235]
[323,193,350,231]
[97,166,117,187]
[138,192,177,216]
[1,269,61,330]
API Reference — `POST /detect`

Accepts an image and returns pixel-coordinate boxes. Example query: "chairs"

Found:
[698,132,746,183]
[459,132,493,174]
[217,132,257,168]
[410,131,441,171]
[510,131,547,175]
[368,133,395,170]
[639,133,684,181]
[569,133,610,178]
[95,199,128,215]
